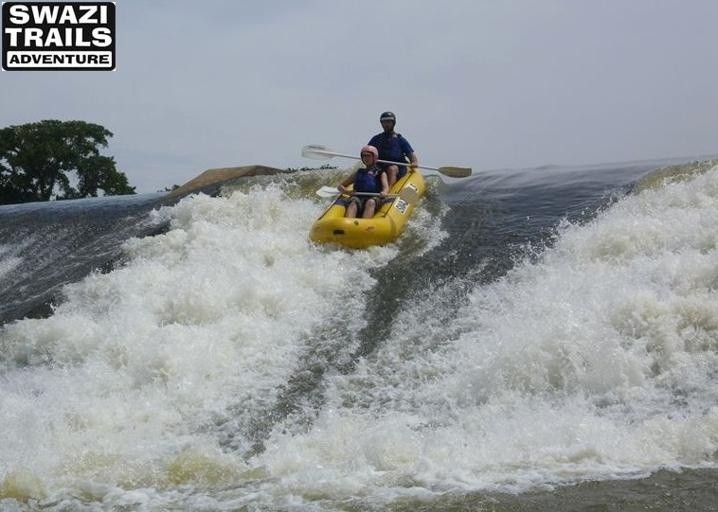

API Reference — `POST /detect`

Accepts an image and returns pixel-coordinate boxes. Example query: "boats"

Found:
[310,163,426,249]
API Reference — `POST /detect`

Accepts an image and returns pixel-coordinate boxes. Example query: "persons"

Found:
[366,111,418,193]
[336,143,389,220]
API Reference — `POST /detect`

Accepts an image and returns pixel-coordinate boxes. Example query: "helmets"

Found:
[379,111,396,125]
[360,145,379,160]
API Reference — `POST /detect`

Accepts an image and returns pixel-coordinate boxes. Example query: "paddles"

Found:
[315,185,418,203]
[301,143,472,178]
